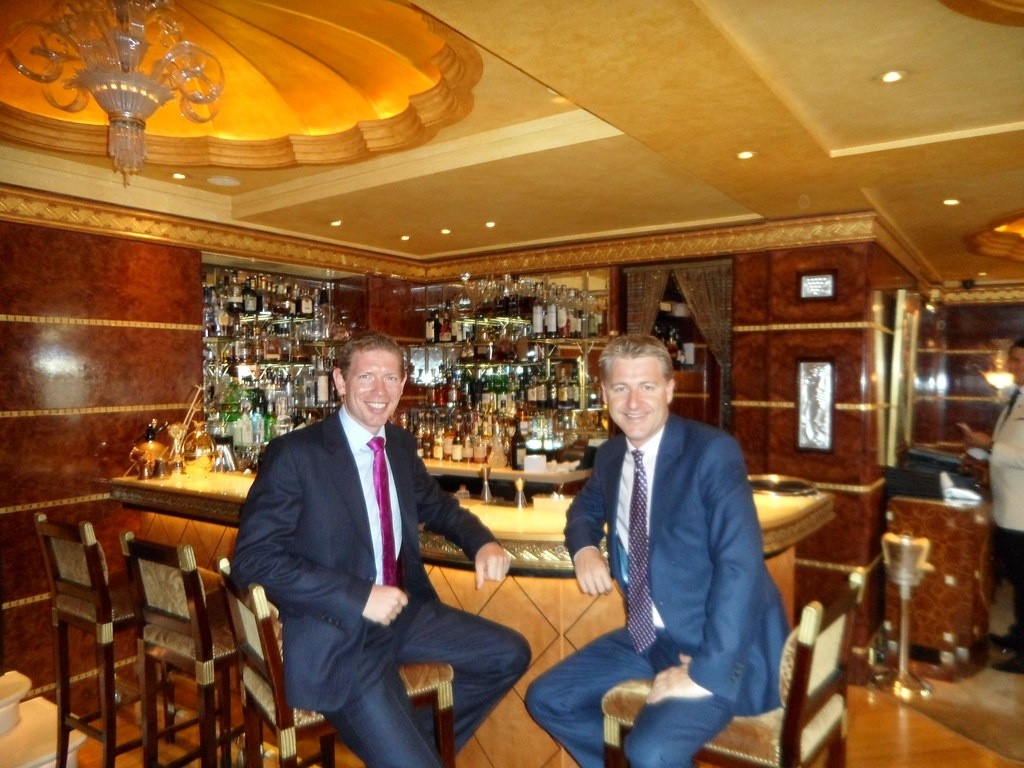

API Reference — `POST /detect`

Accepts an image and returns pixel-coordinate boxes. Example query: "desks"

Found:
[887,495,996,681]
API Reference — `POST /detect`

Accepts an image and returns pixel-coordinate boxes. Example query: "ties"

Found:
[366,435,397,587]
[626,449,657,654]
[1005,388,1021,420]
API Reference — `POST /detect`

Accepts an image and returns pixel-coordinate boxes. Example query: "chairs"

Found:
[119,527,244,768]
[34,511,176,768]
[602,570,864,768]
[217,553,457,768]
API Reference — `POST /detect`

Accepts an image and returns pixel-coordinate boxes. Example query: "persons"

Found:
[964,338,1024,673]
[525,333,790,768]
[232,334,532,768]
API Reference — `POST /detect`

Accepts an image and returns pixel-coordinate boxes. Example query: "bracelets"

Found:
[987,441,994,454]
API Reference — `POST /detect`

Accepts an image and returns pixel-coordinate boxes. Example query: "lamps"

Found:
[4,0,225,185]
[984,338,1016,391]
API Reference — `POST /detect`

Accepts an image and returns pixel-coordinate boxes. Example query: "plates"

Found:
[748,474,815,496]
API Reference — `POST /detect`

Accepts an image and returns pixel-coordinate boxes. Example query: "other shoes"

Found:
[992,656,1024,673]
[989,632,1011,651]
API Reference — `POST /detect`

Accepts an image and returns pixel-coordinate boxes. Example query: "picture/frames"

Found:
[795,355,838,456]
[792,269,838,304]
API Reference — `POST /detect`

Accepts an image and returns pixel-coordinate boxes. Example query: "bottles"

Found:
[481,466,492,501]
[400,272,598,470]
[201,269,341,446]
[513,477,527,508]
